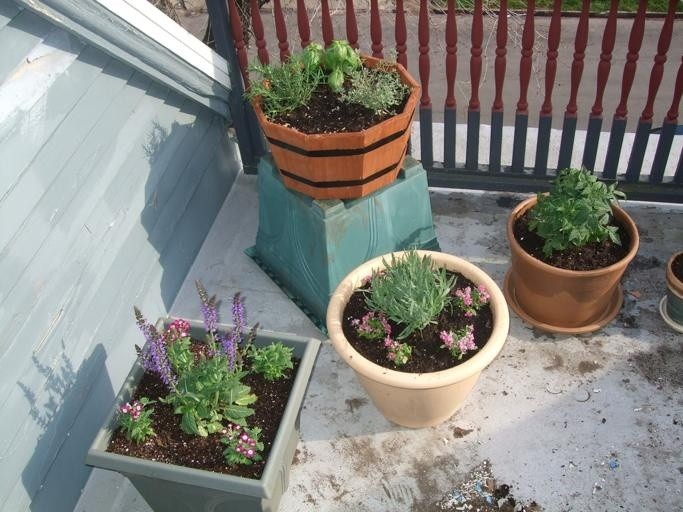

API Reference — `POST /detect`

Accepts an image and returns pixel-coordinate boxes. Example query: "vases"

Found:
[658,251,683,333]
[86,316,321,512]
[324,249,510,425]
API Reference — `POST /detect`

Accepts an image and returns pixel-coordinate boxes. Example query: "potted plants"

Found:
[238,40,422,200]
[503,163,639,336]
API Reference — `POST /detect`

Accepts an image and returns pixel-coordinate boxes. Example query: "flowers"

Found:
[348,251,490,367]
[112,276,298,469]
